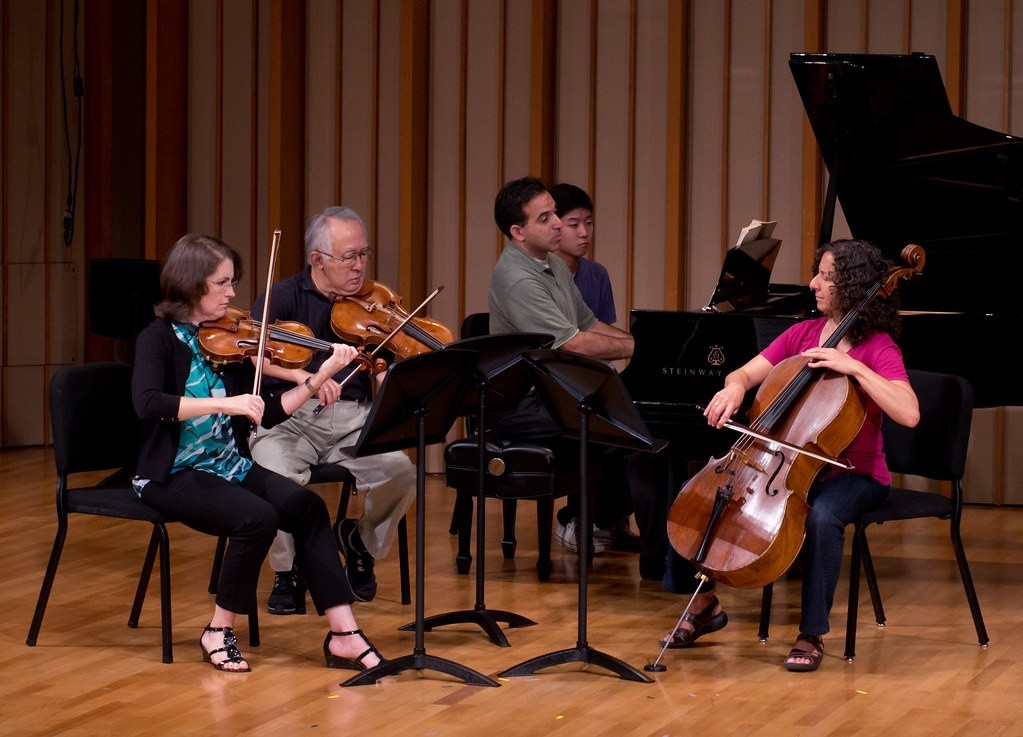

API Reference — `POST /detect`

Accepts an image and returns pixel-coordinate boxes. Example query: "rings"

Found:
[715,402,719,406]
[324,389,329,391]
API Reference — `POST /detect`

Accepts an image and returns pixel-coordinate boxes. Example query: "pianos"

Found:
[620,50,1022,582]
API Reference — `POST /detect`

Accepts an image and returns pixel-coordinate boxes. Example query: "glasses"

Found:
[315,248,375,268]
[207,278,238,293]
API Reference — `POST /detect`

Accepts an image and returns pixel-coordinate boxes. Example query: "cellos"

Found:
[644,242,928,671]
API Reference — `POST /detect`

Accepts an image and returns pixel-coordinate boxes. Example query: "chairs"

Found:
[26,359,261,665]
[755,369,988,663]
[208,461,410,604]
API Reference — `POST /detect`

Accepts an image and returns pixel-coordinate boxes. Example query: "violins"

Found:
[196,303,389,378]
[330,280,454,361]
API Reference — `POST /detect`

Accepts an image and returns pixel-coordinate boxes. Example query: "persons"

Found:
[490,176,635,437]
[249,206,417,614]
[554,182,638,551]
[132,233,398,676]
[659,240,920,671]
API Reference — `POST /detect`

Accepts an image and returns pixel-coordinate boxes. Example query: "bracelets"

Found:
[305,377,321,394]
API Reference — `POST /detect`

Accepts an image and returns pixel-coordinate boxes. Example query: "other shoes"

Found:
[595,525,641,552]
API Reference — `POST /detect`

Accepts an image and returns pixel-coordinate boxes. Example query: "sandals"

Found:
[199,622,252,673]
[659,596,728,647]
[783,634,824,671]
[324,629,398,675]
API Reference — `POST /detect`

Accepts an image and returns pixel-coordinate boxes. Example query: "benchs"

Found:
[445,434,607,583]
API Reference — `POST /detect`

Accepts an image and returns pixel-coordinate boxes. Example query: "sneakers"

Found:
[553,518,605,553]
[266,564,298,613]
[334,519,377,601]
[592,523,611,545]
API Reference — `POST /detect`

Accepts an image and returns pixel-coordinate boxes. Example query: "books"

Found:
[736,219,778,244]
